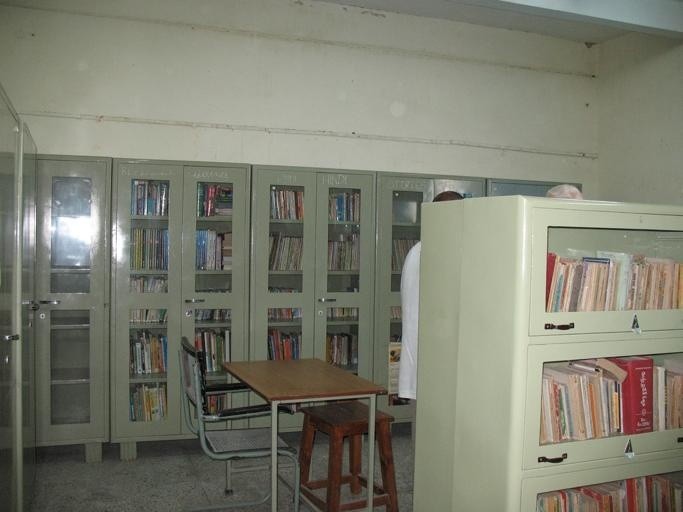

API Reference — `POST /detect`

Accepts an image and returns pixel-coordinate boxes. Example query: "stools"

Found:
[299,400,401,512]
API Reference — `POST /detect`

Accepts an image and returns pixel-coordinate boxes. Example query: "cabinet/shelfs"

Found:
[111,154,251,463]
[419,193,683,512]
[253,161,377,443]
[486,175,585,199]
[1,120,37,511]
[33,152,113,465]
[374,170,489,441]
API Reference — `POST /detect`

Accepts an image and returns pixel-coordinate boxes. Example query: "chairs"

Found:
[175,333,302,510]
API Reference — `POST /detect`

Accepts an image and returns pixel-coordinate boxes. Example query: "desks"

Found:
[219,356,385,510]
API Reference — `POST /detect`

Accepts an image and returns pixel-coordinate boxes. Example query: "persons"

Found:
[398,191,465,461]
[545,184,582,199]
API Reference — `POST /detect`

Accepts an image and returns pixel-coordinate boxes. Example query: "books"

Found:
[535,249,683,512]
[390,240,420,391]
[194,181,233,417]
[327,191,360,366]
[269,185,304,360]
[130,181,168,421]
[286,404,296,412]
